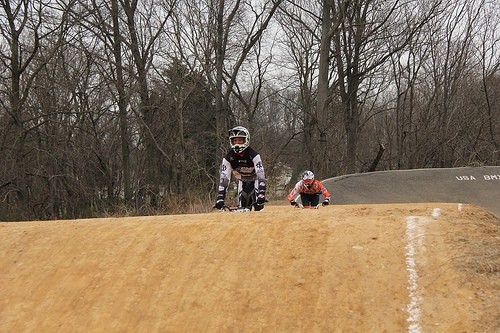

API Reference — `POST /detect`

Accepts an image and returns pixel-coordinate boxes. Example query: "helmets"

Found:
[229,126,250,154]
[301,171,315,190]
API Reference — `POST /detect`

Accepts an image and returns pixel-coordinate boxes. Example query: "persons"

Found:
[213,126,267,211]
[286,170,331,209]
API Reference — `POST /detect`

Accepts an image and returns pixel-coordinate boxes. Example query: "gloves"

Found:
[290,200,297,205]
[216,196,224,209]
[257,192,265,206]
[323,197,330,205]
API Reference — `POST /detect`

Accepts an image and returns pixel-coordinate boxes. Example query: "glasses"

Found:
[304,180,313,184]
